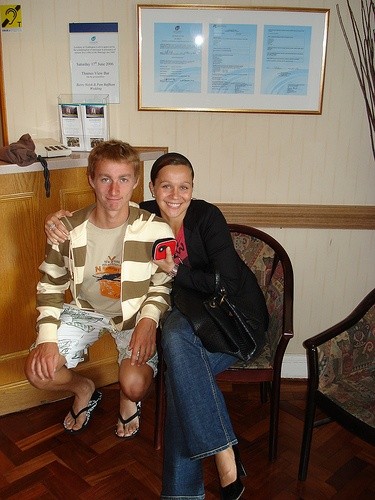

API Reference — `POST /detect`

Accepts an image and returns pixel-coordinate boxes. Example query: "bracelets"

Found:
[167,265,179,277]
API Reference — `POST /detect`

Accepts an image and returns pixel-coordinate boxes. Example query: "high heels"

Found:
[218,447,248,500]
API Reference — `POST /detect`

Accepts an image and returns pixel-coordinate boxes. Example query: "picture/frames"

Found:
[136,3,330,115]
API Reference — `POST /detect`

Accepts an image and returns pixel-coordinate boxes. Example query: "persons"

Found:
[23,140,177,439]
[43,152,269,500]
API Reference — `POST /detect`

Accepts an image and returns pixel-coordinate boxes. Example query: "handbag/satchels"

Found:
[204,269,265,363]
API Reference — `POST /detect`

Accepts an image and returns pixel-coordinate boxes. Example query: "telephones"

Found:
[30,138,72,159]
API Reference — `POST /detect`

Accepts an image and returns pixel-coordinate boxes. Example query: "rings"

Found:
[48,224,55,231]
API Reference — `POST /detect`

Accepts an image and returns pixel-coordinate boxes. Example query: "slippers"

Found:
[60,390,102,432]
[112,395,142,438]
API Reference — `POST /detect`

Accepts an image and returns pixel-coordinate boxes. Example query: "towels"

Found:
[0,133,38,167]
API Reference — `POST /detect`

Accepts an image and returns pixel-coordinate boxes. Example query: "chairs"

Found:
[297,288,375,483]
[155,223,294,461]
